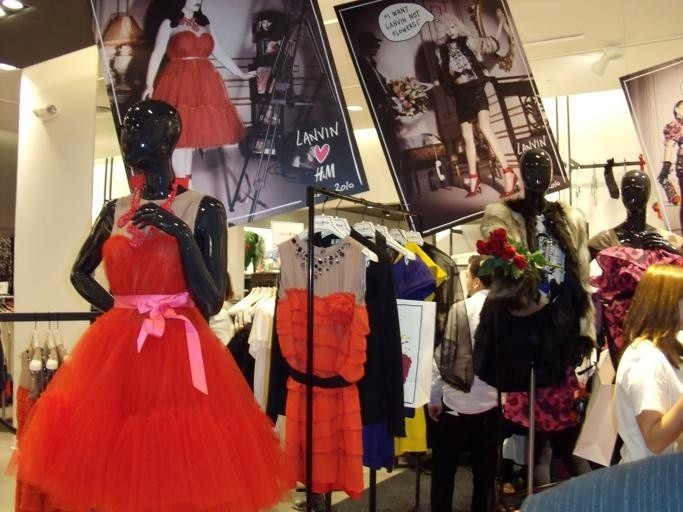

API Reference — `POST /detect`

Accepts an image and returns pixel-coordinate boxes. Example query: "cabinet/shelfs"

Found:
[239,10,293,160]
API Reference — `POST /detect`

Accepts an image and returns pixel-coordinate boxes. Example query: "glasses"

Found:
[369,43,380,49]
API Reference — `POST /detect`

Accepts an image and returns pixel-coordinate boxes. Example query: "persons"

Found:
[656,100,683,235]
[613,264,683,464]
[6,99,297,512]
[427,255,502,511]
[435,14,518,198]
[588,170,683,372]
[209,272,237,345]
[473,147,596,392]
[141,0,261,191]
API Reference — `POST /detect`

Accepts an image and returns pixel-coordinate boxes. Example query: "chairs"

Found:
[452,118,503,188]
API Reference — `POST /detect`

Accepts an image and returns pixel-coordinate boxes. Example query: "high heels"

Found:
[465,174,482,197]
[499,166,519,198]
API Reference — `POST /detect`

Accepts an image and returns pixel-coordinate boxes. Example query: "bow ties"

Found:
[370,59,377,68]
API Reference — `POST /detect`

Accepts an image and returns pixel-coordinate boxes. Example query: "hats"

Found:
[358,32,382,42]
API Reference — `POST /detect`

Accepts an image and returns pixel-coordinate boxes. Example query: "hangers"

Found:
[0,297,14,313]
[228,271,279,316]
[26,312,42,371]
[267,186,424,263]
[54,312,71,364]
[42,312,59,370]
[574,154,645,206]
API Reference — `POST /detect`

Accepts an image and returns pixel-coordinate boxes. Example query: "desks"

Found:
[405,143,452,198]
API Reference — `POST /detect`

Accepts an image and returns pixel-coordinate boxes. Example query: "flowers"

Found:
[476,227,564,280]
[388,75,430,139]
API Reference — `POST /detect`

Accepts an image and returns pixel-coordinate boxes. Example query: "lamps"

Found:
[100,15,150,92]
